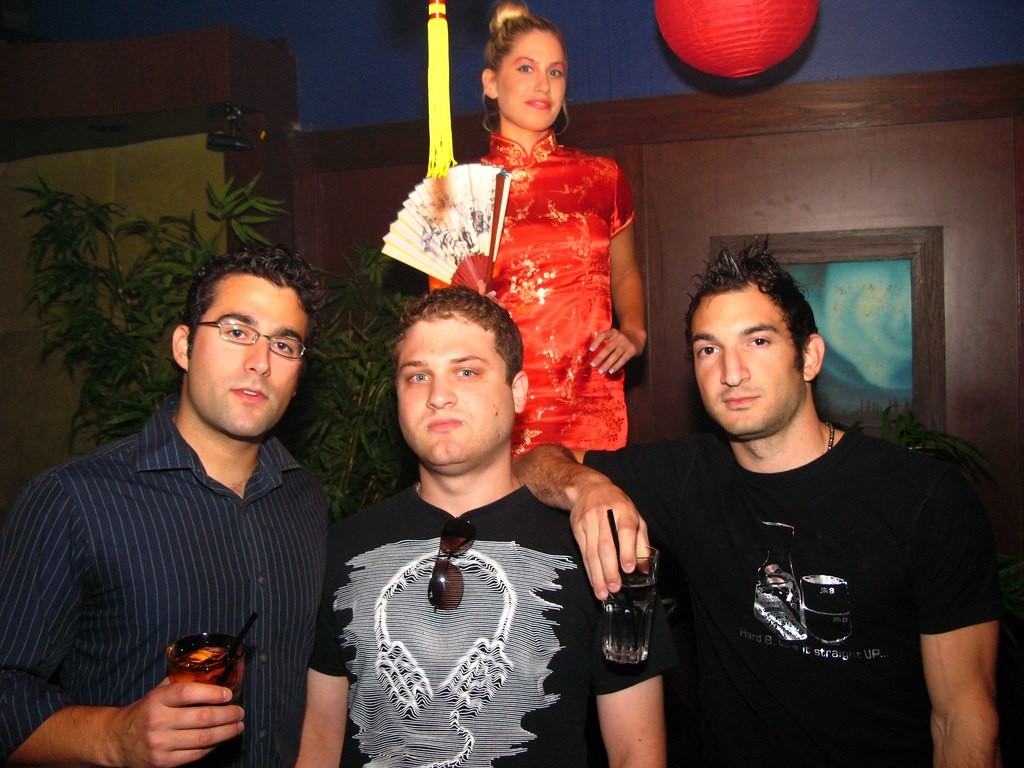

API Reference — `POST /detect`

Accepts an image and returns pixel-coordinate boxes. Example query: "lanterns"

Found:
[653,0,821,78]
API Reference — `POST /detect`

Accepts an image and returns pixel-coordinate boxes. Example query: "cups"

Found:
[602,544,659,663]
[165,632,247,768]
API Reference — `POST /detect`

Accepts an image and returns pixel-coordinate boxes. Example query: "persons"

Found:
[427,0,648,455]
[0,243,327,768]
[291,287,679,768]
[510,234,1000,768]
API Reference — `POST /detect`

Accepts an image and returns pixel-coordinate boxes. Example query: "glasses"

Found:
[427,518,477,612]
[182,321,308,359]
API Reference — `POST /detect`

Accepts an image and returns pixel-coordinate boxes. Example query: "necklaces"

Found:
[825,423,834,450]
